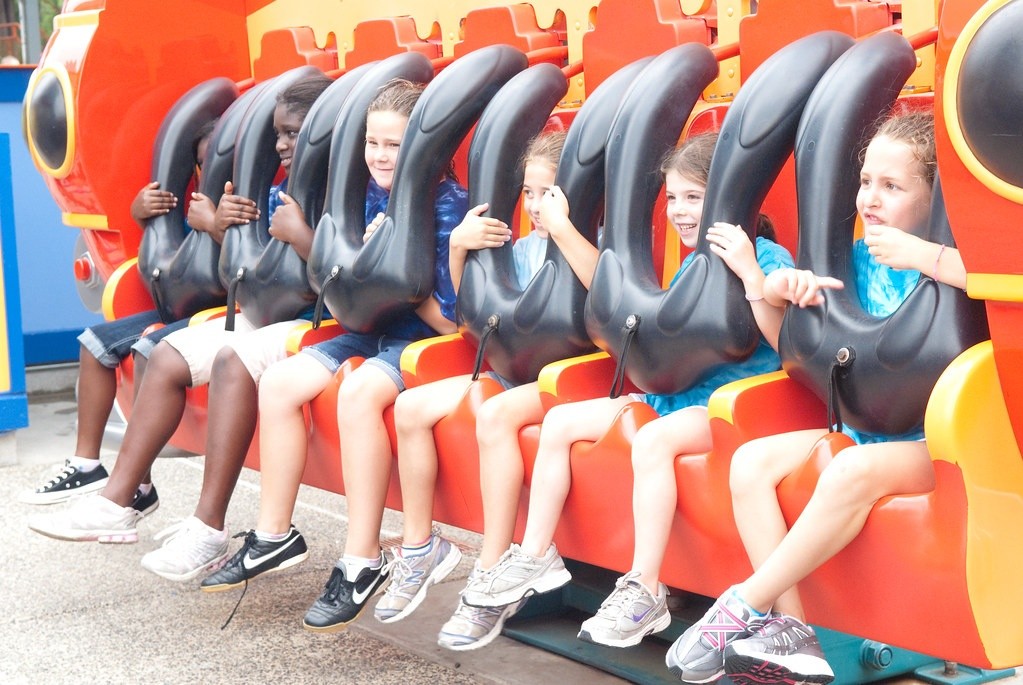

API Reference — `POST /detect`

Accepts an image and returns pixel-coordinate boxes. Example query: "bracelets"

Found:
[745,295,766,302]
[934,244,945,280]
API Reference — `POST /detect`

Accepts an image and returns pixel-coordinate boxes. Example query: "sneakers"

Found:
[436,556,530,652]
[198,524,309,631]
[28,495,145,544]
[131,484,160,517]
[302,545,392,634]
[373,533,463,625]
[18,457,110,506]
[721,615,835,685]
[666,585,772,685]
[578,571,671,649]
[138,516,233,583]
[458,540,574,609]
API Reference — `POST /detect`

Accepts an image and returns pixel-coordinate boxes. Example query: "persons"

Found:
[27,117,225,520]
[198,79,474,633]
[462,132,796,648]
[29,76,342,581]
[665,113,969,685]
[374,132,605,648]
